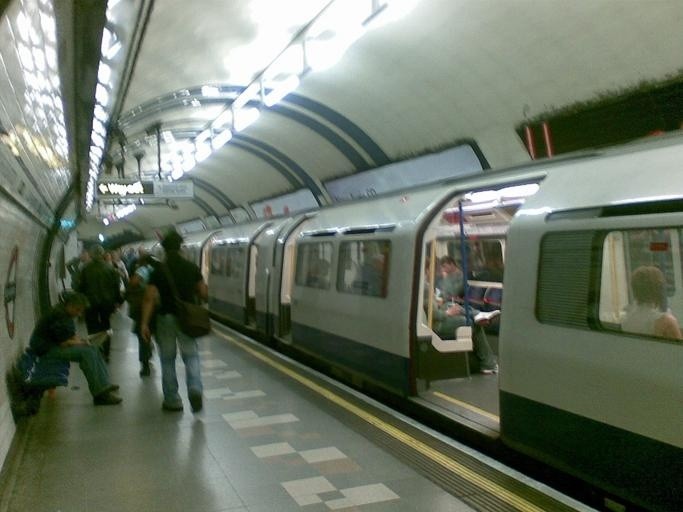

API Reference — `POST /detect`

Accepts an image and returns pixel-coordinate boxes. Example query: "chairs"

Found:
[462,284,502,335]
[11,346,71,420]
[423,310,473,386]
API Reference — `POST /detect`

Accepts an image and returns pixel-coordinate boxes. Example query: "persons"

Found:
[28,223,210,413]
[619,265,682,340]
[305,241,502,376]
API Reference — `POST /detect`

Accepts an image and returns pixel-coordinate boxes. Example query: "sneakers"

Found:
[163,398,184,411]
[480,365,498,373]
[474,309,501,327]
[140,368,150,376]
[190,388,203,411]
[106,385,119,392]
[93,392,123,405]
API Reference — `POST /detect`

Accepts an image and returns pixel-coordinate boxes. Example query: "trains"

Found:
[116,127,682,512]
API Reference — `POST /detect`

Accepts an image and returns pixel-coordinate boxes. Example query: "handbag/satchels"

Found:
[175,299,212,338]
